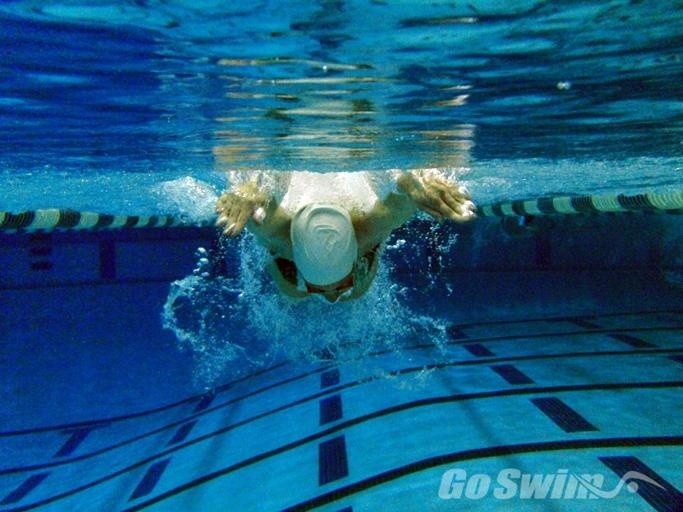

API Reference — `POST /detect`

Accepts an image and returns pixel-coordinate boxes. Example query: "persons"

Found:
[215,171,478,303]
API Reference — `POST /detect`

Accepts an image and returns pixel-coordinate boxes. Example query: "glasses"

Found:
[304,276,354,292]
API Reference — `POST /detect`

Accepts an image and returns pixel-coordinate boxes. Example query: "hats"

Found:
[290,202,357,287]
